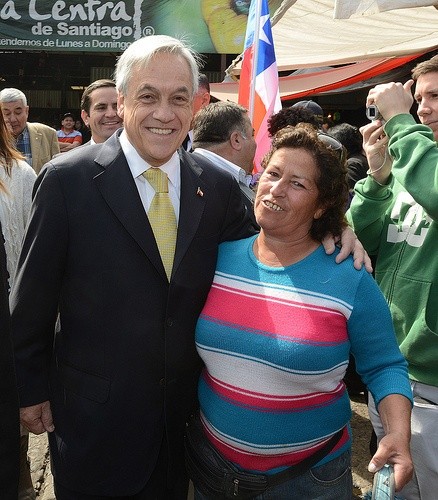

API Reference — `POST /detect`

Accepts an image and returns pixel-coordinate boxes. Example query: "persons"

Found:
[195,124,413,500]
[0,75,366,202]
[12,35,373,500]
[0,101,38,490]
[349,55,438,500]
[0,222,19,500]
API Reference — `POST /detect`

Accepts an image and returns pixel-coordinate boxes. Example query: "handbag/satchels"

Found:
[188,430,345,500]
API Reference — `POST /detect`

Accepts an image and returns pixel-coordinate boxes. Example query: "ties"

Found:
[141,167,178,284]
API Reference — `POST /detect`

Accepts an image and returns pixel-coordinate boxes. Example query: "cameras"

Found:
[366,104,388,136]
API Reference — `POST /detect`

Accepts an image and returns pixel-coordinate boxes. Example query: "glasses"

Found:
[276,126,343,171]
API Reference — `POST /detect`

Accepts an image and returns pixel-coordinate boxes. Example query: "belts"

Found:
[413,383,438,404]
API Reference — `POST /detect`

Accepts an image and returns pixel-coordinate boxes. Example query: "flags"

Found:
[239,1,284,177]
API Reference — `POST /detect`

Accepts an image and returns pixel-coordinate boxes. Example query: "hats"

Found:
[288,100,324,115]
